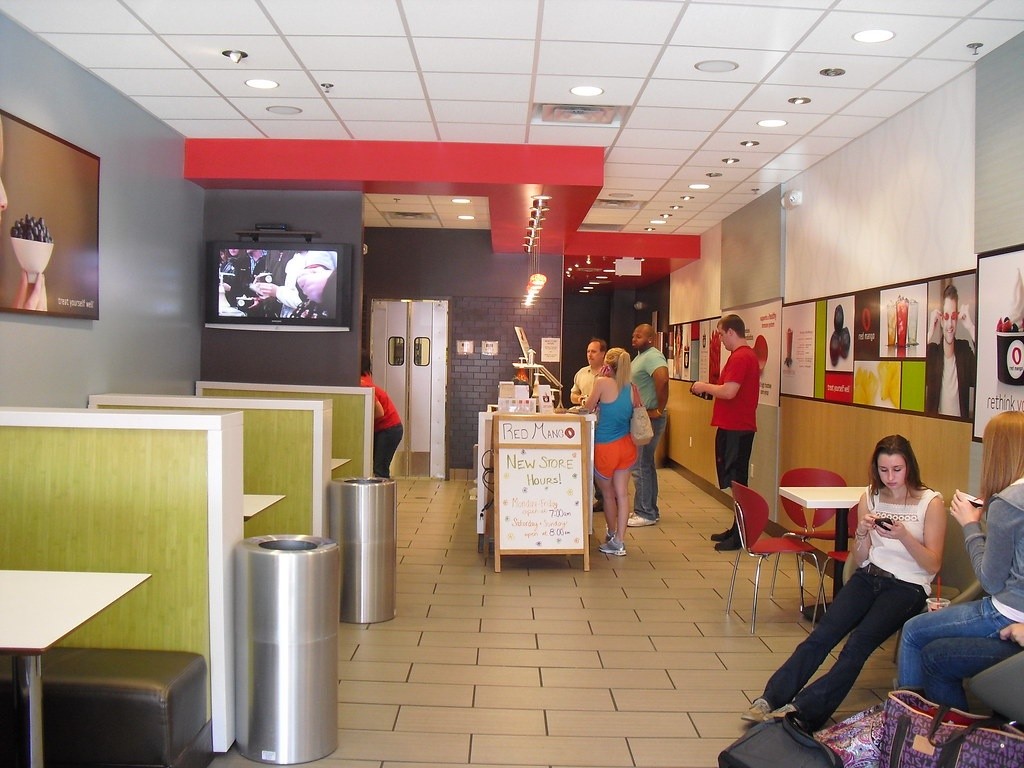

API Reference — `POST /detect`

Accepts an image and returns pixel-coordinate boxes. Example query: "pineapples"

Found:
[854,360,901,408]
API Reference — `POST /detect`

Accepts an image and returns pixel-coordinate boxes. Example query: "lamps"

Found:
[521,195,551,306]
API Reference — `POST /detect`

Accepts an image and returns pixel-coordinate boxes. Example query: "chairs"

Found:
[725,468,859,634]
[941,575,1023,726]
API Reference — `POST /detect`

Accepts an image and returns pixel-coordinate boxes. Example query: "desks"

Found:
[0,569,153,768]
[778,486,867,624]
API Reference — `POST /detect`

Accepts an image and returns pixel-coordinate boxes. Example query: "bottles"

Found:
[745,329,754,347]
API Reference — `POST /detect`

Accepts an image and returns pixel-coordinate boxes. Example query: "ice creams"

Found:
[995,267,1024,385]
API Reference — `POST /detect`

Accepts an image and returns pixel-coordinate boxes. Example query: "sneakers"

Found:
[606,529,615,540]
[599,538,626,555]
[741,698,771,721]
[763,703,796,721]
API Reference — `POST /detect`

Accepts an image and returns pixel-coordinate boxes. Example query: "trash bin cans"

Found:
[234,534,339,765]
[328,477,396,623]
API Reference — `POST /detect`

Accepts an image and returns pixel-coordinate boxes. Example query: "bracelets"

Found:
[657,409,663,415]
[854,530,869,551]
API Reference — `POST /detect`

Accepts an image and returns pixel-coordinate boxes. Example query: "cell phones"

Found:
[874,517,893,532]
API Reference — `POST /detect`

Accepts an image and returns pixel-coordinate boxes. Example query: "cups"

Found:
[886,303,897,345]
[684,351,689,368]
[703,335,706,347]
[908,301,918,345]
[950,493,983,516]
[895,300,909,348]
[10,236,54,284]
[927,597,951,613]
[787,332,793,359]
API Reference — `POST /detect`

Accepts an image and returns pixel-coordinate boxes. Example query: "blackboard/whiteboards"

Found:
[485,415,590,555]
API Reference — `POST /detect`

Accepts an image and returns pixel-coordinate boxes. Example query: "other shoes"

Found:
[593,501,604,511]
[715,531,742,550]
[711,519,738,541]
[629,513,659,520]
[627,516,655,527]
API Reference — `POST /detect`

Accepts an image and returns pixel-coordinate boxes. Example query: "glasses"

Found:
[943,312,958,320]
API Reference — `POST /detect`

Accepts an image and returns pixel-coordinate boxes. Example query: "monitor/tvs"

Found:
[203,241,354,332]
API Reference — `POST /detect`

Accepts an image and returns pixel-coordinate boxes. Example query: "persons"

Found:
[361,351,403,478]
[0,115,47,311]
[628,324,669,526]
[741,433,947,728]
[586,348,638,556]
[926,285,975,416]
[220,249,337,319]
[693,313,759,551]
[895,410,1024,727]
[571,339,617,511]
[674,325,683,379]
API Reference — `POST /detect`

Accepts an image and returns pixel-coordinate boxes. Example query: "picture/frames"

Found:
[0,109,100,320]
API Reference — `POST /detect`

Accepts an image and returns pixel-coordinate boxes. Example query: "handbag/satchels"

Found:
[630,383,653,446]
[880,690,1023,768]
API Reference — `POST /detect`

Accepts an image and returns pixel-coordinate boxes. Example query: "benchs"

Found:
[0,646,212,768]
